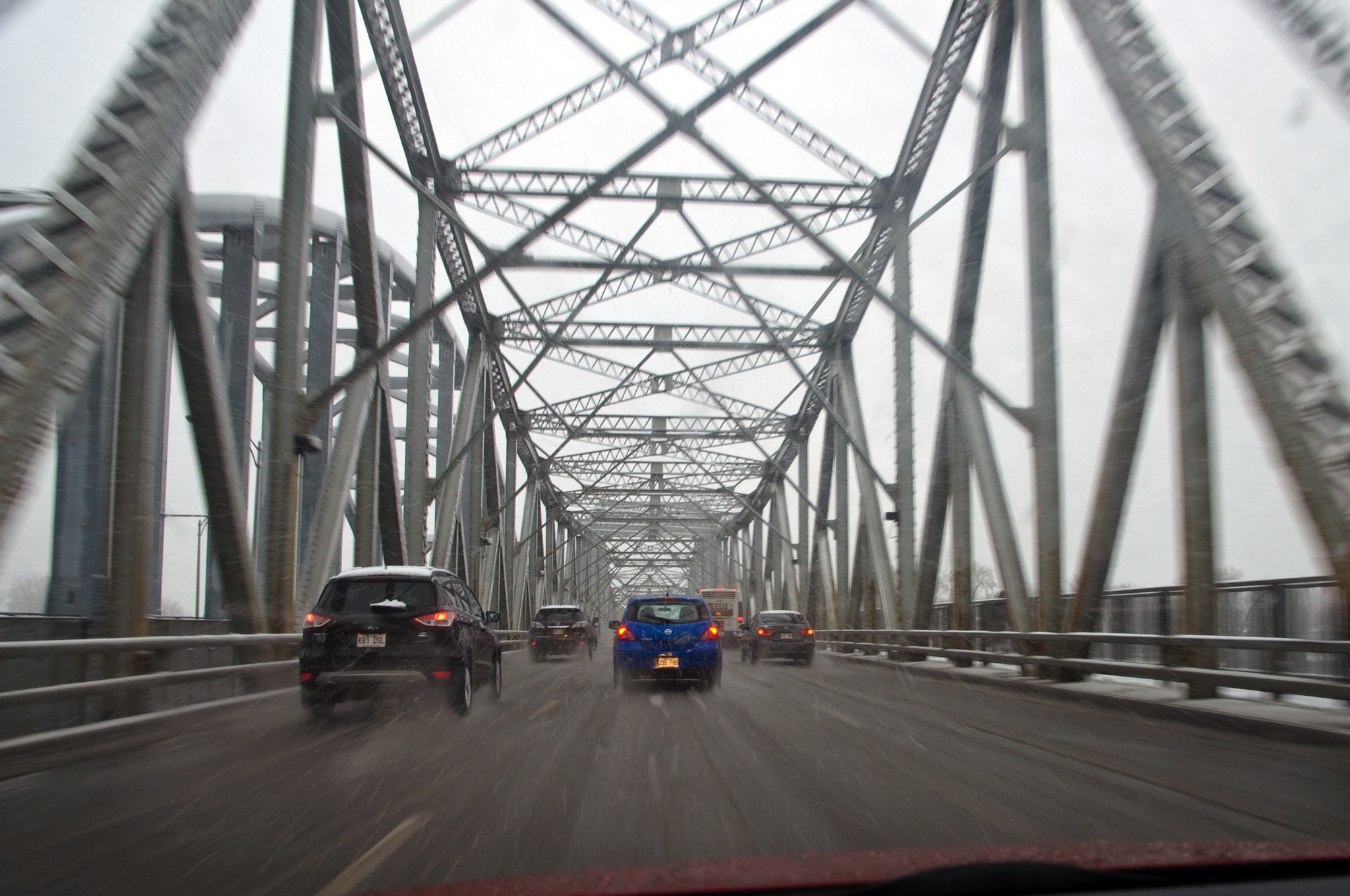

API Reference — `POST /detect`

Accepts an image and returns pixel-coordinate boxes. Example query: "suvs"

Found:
[301,561,506,717]
[528,605,600,662]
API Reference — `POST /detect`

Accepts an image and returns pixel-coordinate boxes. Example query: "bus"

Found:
[697,589,738,644]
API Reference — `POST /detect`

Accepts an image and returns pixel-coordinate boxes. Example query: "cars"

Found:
[608,584,722,688]
[739,610,815,665]
